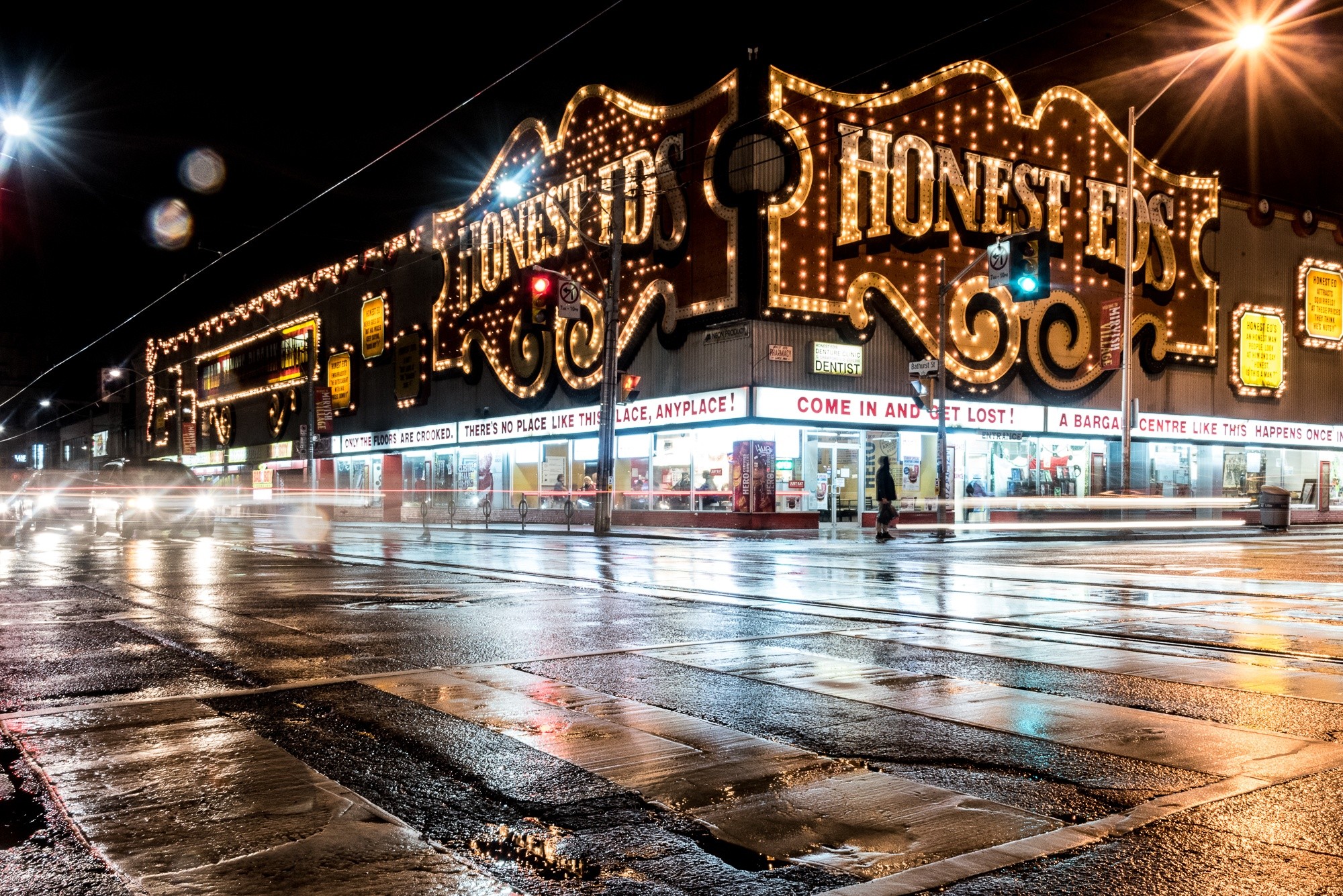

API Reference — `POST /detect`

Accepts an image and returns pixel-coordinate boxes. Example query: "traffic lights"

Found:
[622,375,642,404]
[1009,233,1052,301]
[912,378,935,410]
[522,273,553,332]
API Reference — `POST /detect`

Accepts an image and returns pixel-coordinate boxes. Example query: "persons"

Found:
[550,466,732,516]
[873,455,896,544]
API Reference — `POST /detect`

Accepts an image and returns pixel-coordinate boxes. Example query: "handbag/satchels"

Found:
[878,503,899,524]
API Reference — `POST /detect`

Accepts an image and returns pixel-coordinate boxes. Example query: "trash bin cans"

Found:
[1261,484,1291,532]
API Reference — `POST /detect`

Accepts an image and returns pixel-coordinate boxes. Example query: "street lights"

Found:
[1113,22,1272,479]
[110,367,183,462]
[40,398,95,487]
[532,262,611,536]
[238,307,318,486]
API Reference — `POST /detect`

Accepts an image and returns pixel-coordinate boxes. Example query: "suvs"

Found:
[86,457,217,538]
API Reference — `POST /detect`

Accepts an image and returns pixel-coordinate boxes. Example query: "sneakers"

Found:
[882,532,896,540]
[875,533,884,541]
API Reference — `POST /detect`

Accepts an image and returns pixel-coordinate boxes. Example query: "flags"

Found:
[180,422,198,456]
[315,387,334,434]
[1097,298,1123,370]
